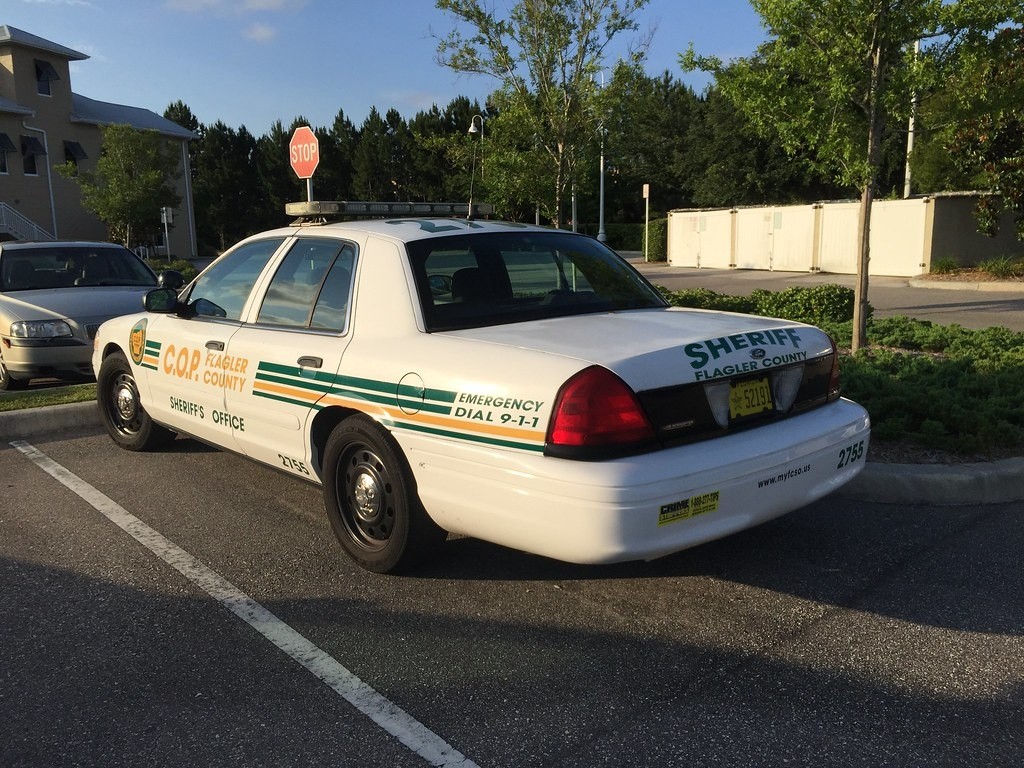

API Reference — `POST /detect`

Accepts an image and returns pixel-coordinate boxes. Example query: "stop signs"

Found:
[287,128,318,180]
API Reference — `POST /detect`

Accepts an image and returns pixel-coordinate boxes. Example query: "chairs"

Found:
[313,265,350,308]
[75,257,109,286]
[451,267,491,308]
[6,260,37,288]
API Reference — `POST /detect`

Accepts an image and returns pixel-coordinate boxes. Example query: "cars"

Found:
[0,242,182,392]
[90,201,869,575]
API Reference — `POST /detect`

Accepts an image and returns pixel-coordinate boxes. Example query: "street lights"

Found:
[469,115,484,183]
[589,70,606,242]
